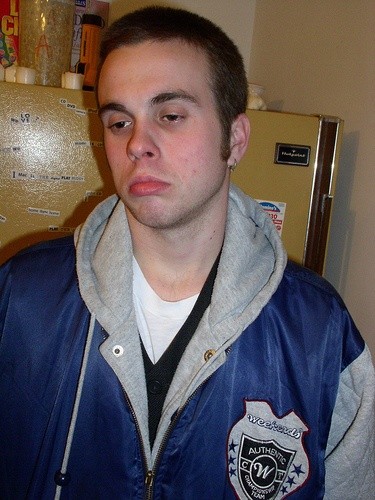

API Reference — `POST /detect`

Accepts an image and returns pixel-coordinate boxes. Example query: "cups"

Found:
[247,82,264,110]
[16,68,36,84]
[6,67,16,82]
[64,73,85,90]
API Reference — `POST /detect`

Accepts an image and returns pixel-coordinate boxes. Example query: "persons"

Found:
[0,6,375,500]
